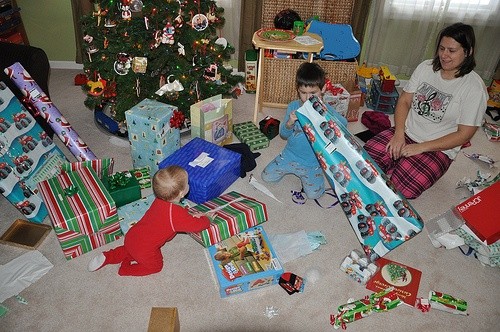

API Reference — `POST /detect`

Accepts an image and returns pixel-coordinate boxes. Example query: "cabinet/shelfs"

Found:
[369,79,399,114]
[0,0,29,46]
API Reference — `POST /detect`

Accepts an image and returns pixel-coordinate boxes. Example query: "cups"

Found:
[293,20,304,37]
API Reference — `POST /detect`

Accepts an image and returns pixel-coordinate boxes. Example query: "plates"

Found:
[256,28,296,42]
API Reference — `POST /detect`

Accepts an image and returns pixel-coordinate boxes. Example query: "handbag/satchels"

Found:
[451,179,500,267]
[189,94,234,147]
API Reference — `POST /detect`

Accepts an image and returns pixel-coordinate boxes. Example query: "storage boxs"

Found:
[0,82,71,224]
[454,179,500,245]
[147,307,181,332]
[98,171,142,208]
[245,49,258,94]
[324,90,362,123]
[366,258,423,306]
[339,250,380,286]
[38,166,124,261]
[125,98,283,297]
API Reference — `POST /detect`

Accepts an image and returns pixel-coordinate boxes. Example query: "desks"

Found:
[253,28,324,122]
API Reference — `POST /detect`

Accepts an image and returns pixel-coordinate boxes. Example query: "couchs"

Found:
[0,43,55,140]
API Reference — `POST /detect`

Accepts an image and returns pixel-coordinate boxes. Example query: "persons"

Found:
[363,22,490,199]
[0,42,54,139]
[261,62,348,200]
[88,166,218,276]
